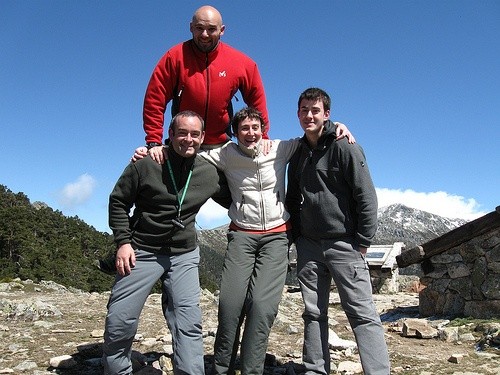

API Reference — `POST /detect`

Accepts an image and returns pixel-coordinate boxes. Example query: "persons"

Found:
[143,5,272,165]
[130,108,356,375]
[284,88,390,375]
[102,113,232,375]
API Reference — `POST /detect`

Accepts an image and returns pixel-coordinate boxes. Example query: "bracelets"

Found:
[146,142,160,149]
